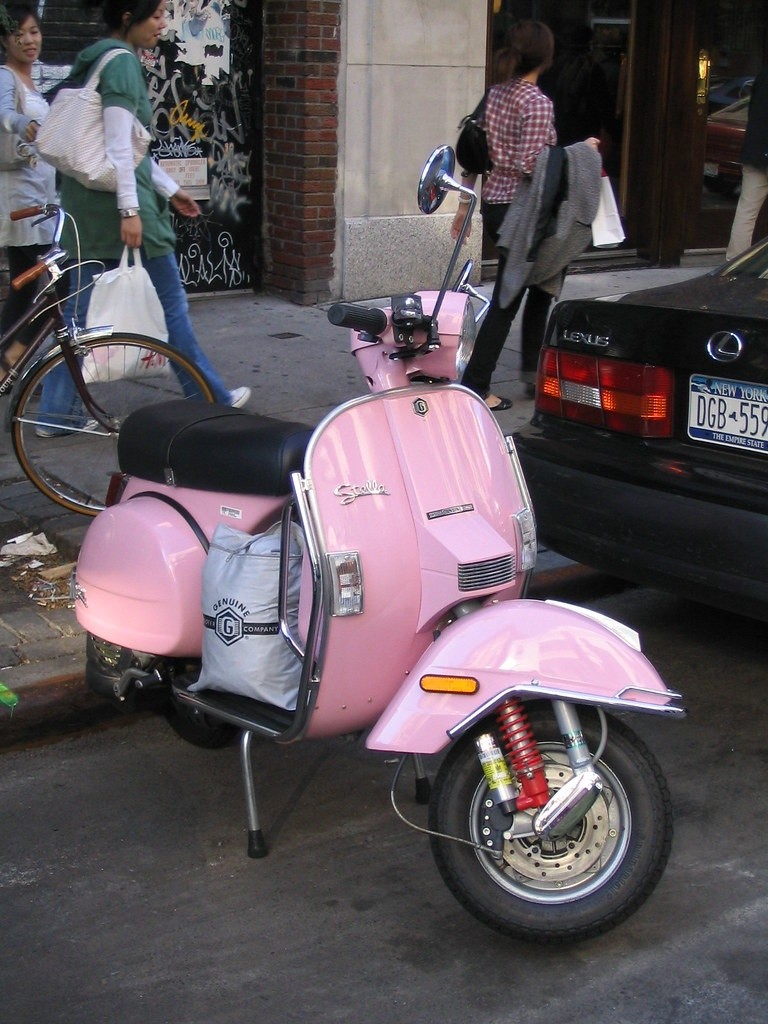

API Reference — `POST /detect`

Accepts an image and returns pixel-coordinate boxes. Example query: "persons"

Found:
[0,0,56,395]
[36,0,251,438]
[449,19,600,411]
[726,67,768,261]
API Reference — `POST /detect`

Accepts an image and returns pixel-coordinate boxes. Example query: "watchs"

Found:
[121,209,138,218]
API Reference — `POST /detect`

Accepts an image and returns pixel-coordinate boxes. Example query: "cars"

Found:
[707,76,756,193]
[508,236,767,624]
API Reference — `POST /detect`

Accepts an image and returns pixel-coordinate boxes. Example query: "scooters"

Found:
[76,145,686,945]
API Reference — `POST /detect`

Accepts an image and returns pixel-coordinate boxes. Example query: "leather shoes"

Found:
[525,384,536,399]
[483,396,512,410]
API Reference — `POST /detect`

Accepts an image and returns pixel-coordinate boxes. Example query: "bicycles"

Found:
[2,204,214,517]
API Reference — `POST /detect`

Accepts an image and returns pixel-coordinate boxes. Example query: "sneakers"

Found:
[30,417,99,440]
[228,385,252,412]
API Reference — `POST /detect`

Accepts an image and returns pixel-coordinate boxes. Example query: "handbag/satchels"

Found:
[592,168,625,250]
[33,47,152,194]
[0,63,36,171]
[185,520,308,712]
[456,90,493,175]
[78,245,170,385]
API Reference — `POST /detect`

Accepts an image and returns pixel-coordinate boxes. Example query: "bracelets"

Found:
[458,196,471,204]
